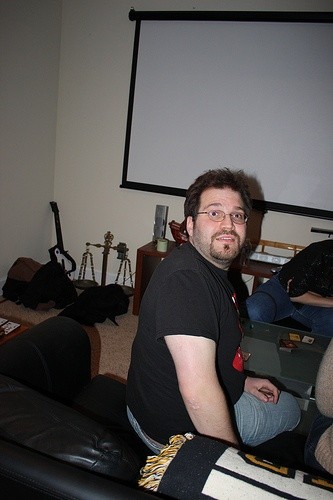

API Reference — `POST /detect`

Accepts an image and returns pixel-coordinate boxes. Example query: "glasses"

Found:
[196,210,248,224]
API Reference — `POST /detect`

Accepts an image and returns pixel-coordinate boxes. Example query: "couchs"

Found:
[0,317,333,500]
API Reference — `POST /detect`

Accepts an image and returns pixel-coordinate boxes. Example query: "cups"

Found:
[156,239,168,252]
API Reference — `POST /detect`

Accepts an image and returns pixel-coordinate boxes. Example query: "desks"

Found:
[239,318,333,399]
[132,240,284,325]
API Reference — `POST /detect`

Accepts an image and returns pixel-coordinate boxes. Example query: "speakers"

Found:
[153,205,168,239]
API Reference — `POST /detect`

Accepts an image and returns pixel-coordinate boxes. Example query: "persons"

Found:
[126,166,302,457]
[313,334,333,480]
[245,237,333,332]
